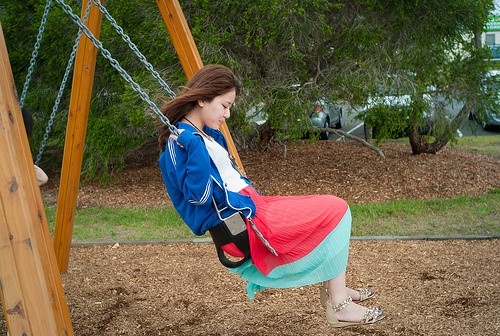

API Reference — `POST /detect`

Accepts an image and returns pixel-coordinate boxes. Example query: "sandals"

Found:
[319,281,376,311]
[325,295,388,328]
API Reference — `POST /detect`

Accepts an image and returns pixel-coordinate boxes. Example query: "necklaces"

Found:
[181,115,215,142]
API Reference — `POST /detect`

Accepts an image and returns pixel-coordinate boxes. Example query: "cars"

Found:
[311,98,345,140]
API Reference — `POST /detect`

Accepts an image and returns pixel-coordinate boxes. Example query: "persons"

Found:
[18,101,48,190]
[156,65,387,328]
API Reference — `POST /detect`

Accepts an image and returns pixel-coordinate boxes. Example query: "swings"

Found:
[54,0,251,269]
